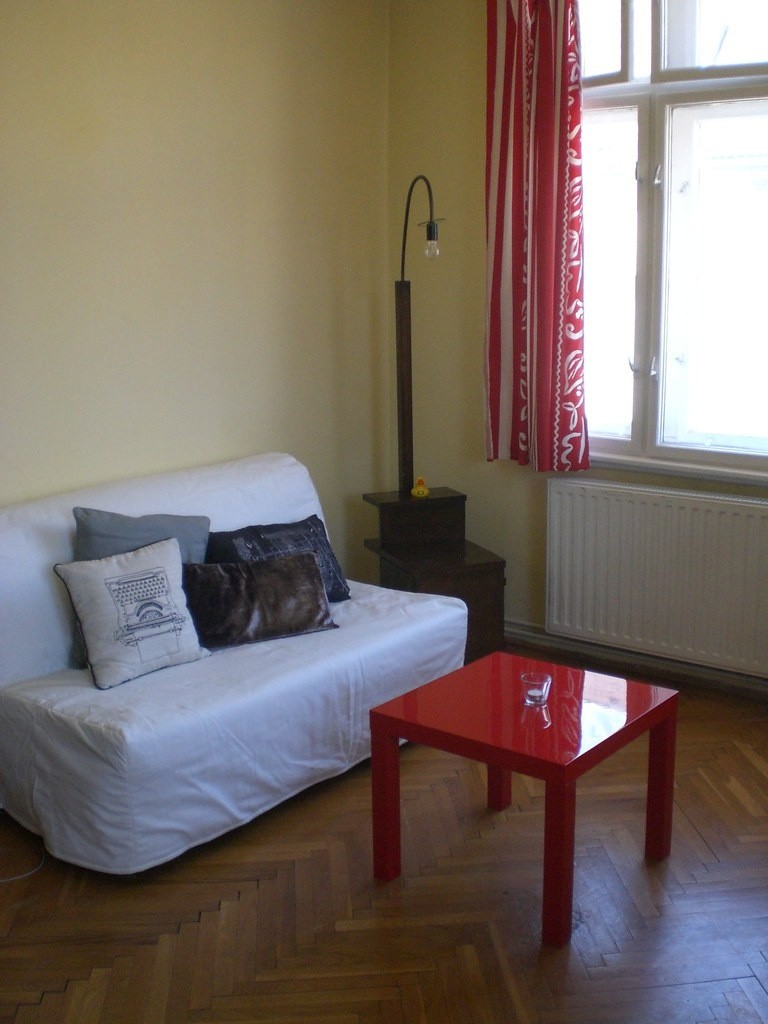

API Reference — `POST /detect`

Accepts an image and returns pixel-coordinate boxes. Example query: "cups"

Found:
[521,672,552,705]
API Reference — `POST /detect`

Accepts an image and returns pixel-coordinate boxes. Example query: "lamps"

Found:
[394,173,446,491]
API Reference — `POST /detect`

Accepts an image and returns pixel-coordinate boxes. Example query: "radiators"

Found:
[544,477,768,681]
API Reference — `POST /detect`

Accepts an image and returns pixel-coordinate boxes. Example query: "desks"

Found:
[370,650,679,949]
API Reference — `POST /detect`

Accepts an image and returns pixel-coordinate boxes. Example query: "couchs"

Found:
[1,451,468,873]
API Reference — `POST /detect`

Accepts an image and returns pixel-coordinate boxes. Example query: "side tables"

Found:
[360,486,507,650]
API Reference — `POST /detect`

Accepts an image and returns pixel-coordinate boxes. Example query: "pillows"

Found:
[206,513,352,603]
[71,507,211,671]
[53,536,211,690]
[182,551,340,653]
